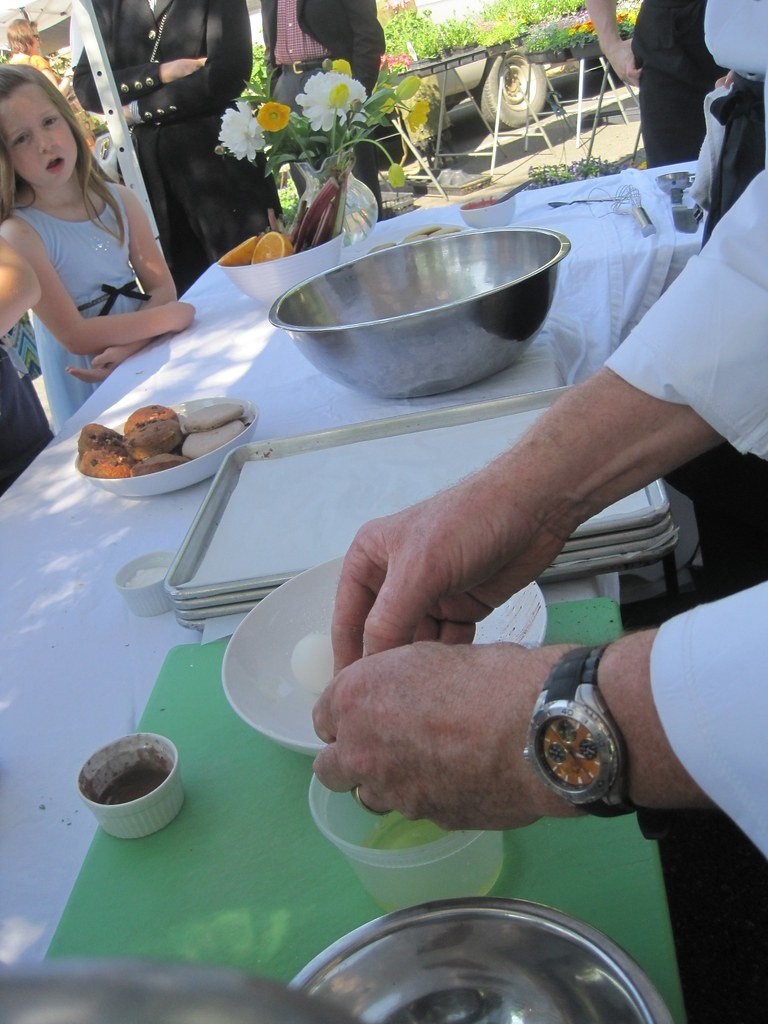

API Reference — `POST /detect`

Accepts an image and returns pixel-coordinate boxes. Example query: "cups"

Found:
[656,172,696,195]
[308,774,505,913]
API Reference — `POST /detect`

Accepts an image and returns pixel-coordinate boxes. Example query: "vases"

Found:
[568,33,626,58]
[413,177,491,196]
[527,48,570,64]
[293,148,377,247]
[380,189,414,221]
[583,107,641,128]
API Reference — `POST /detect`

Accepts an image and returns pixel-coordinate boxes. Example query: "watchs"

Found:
[529,643,637,821]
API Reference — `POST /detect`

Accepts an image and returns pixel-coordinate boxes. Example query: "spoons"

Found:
[548,199,603,208]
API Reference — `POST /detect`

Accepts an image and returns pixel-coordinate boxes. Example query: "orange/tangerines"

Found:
[217,235,257,266]
[251,232,294,265]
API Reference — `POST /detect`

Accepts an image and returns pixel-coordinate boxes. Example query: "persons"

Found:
[260,1,386,222]
[581,0,728,169]
[679,0,768,589]
[70,0,252,295]
[312,160,768,862]
[0,64,196,438]
[0,310,55,497]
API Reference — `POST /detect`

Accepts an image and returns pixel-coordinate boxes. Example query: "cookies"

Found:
[366,225,461,253]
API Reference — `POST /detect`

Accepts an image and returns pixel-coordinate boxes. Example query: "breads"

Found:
[77,403,247,479]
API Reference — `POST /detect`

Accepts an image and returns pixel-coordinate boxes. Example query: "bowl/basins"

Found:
[459,193,516,228]
[114,552,176,616]
[286,897,674,1024]
[76,733,183,838]
[268,227,571,398]
[217,226,346,307]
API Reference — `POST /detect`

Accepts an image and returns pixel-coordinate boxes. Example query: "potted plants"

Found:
[380,0,532,86]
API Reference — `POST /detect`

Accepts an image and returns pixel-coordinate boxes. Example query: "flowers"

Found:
[216,54,430,238]
[521,0,643,54]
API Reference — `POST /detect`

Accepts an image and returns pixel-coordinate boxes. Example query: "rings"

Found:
[355,786,393,816]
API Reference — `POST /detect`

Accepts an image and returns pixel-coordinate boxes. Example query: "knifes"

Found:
[671,188,699,234]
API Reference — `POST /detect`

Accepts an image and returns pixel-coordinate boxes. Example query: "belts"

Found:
[281,57,331,75]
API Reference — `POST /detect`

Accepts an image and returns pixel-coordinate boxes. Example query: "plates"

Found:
[222,553,547,756]
[75,398,260,496]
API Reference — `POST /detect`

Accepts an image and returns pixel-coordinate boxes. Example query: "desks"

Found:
[1,306,688,1024]
[176,160,704,378]
